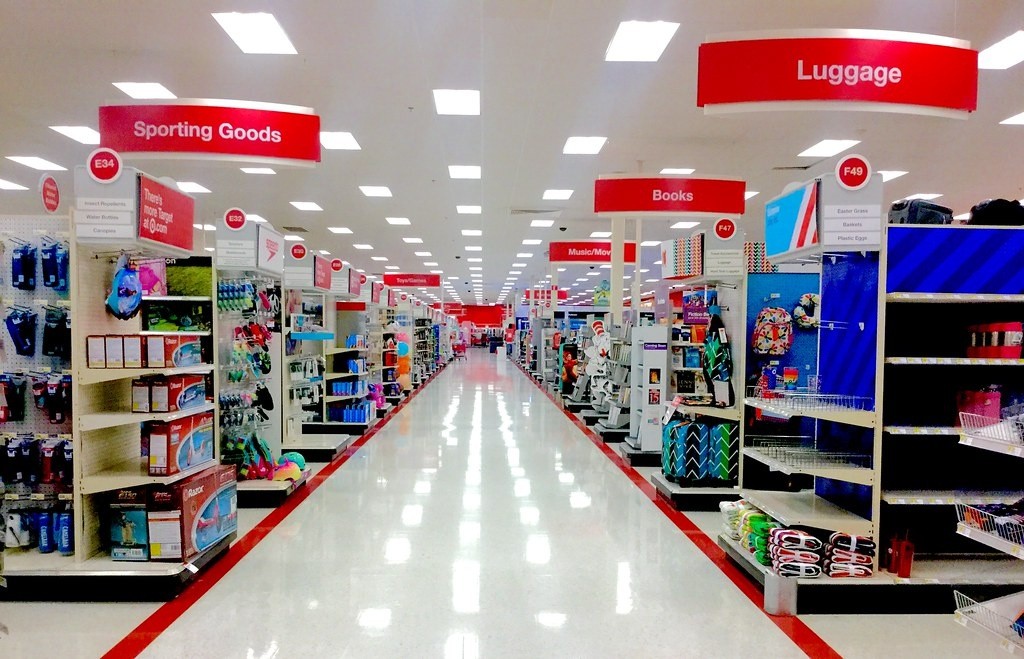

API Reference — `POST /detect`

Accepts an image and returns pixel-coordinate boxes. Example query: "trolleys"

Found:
[451,341,468,361]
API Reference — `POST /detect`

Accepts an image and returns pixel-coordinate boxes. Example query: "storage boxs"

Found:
[146,463,238,563]
[105,335,123,369]
[348,359,363,373]
[123,334,148,369]
[131,378,151,413]
[346,334,364,349]
[148,412,214,477]
[87,335,105,369]
[332,380,367,397]
[111,510,149,562]
[151,376,205,413]
[344,400,376,423]
[148,335,201,368]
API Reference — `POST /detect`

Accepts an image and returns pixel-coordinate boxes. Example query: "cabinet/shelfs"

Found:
[382,308,459,407]
[288,291,379,435]
[503,221,1024,614]
[216,264,311,509]
[0,207,239,602]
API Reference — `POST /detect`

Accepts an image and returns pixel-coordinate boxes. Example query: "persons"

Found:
[476,330,485,339]
[565,353,578,382]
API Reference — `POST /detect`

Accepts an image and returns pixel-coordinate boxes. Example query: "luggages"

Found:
[661,412,739,488]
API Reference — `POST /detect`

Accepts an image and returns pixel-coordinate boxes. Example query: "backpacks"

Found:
[750,307,793,357]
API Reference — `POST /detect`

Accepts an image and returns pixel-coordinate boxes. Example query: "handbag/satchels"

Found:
[702,314,735,407]
